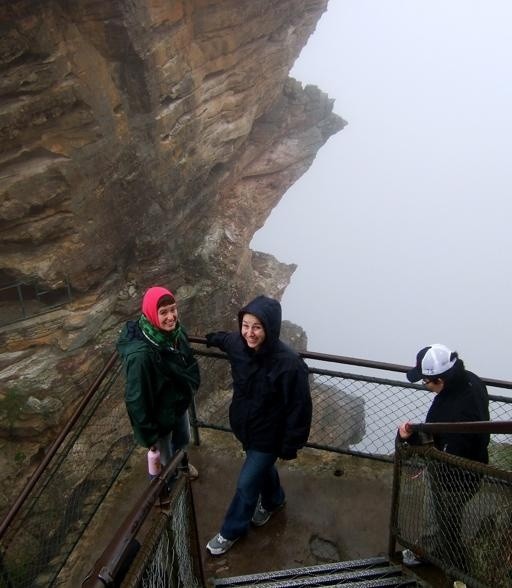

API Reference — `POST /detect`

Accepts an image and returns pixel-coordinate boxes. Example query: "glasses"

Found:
[421,379,436,385]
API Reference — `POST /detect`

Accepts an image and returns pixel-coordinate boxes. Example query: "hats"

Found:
[407,344,457,383]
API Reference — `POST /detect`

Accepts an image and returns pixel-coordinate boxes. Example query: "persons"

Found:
[398,344,491,588]
[115,286,200,499]
[205,295,313,557]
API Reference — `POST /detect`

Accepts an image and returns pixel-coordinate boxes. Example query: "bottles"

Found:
[147,446,161,476]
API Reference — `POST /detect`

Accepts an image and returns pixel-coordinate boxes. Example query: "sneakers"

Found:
[395,549,423,566]
[206,532,240,555]
[252,501,272,525]
[188,464,199,482]
